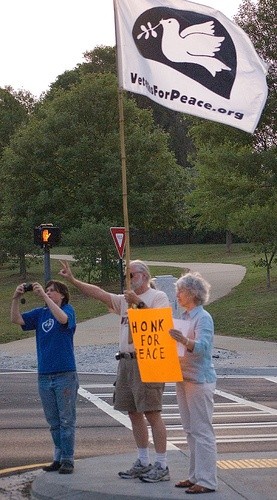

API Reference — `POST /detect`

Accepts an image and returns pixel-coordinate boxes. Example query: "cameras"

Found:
[23,283,33,292]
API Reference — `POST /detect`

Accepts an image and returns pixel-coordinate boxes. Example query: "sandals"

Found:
[185,484,215,494]
[175,479,196,487]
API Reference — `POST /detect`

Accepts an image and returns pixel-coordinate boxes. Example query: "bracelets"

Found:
[183,337,189,347]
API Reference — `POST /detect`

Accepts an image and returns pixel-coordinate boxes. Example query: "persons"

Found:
[10,280,79,474]
[58,259,170,483]
[168,272,218,495]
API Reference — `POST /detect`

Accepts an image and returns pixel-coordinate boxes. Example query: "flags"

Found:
[114,0,272,134]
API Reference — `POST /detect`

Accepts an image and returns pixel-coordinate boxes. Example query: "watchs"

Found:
[137,302,144,308]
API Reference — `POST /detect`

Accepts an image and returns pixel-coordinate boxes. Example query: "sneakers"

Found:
[139,461,170,483]
[118,458,153,479]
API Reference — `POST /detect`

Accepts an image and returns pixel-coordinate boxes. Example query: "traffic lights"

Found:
[40,227,61,245]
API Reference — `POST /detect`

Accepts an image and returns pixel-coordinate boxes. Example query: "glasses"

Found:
[123,272,141,280]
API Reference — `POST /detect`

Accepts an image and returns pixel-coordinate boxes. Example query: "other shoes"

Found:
[59,462,73,473]
[43,461,61,471]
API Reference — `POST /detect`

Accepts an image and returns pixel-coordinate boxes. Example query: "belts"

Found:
[119,352,137,359]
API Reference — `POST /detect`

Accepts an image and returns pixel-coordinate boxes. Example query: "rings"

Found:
[175,335,178,338]
